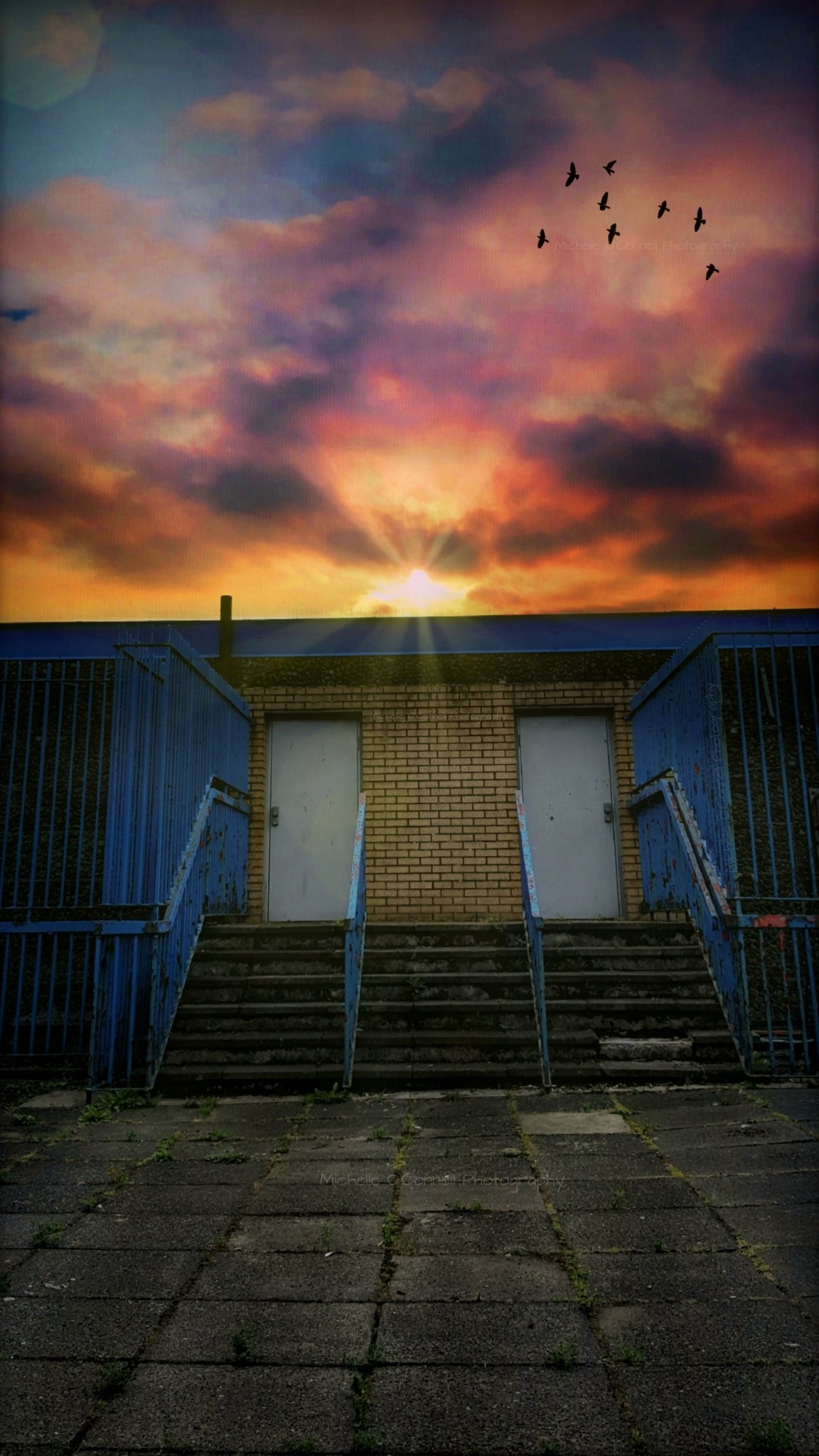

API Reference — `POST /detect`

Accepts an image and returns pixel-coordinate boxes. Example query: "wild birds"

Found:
[537,155,721,284]
